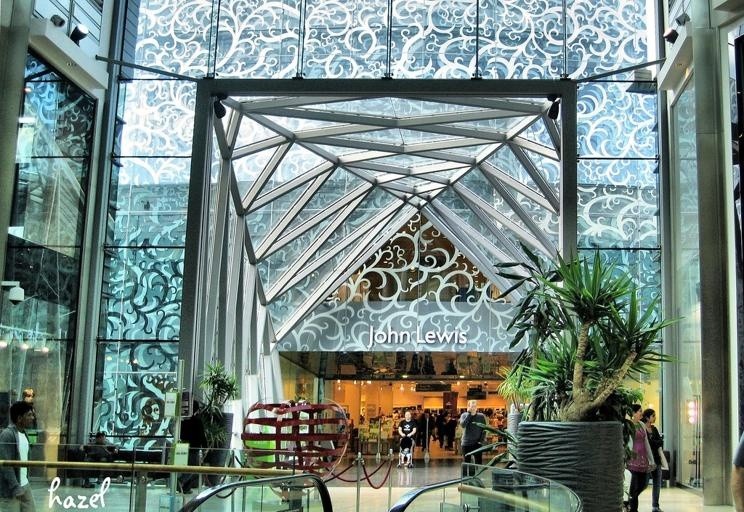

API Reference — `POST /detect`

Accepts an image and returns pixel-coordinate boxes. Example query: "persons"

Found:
[620,402,657,511]
[78,430,120,488]
[397,401,509,454]
[397,410,417,466]
[173,399,208,493]
[637,408,669,511]
[729,431,743,512]
[21,387,37,445]
[0,400,37,512]
[458,399,486,478]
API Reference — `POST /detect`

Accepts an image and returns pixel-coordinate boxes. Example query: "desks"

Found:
[114,450,163,487]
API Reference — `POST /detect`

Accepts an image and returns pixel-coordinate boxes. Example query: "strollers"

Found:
[396,437,416,468]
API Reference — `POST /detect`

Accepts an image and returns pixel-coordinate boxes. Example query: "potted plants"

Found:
[181,358,240,487]
[495,245,684,512]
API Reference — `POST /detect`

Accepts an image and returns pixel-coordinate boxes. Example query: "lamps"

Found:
[663,28,677,43]
[1,281,24,305]
[213,93,228,118]
[70,25,89,41]
[0,326,51,353]
[547,94,560,119]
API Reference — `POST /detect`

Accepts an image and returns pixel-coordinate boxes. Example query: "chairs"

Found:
[83,445,113,484]
